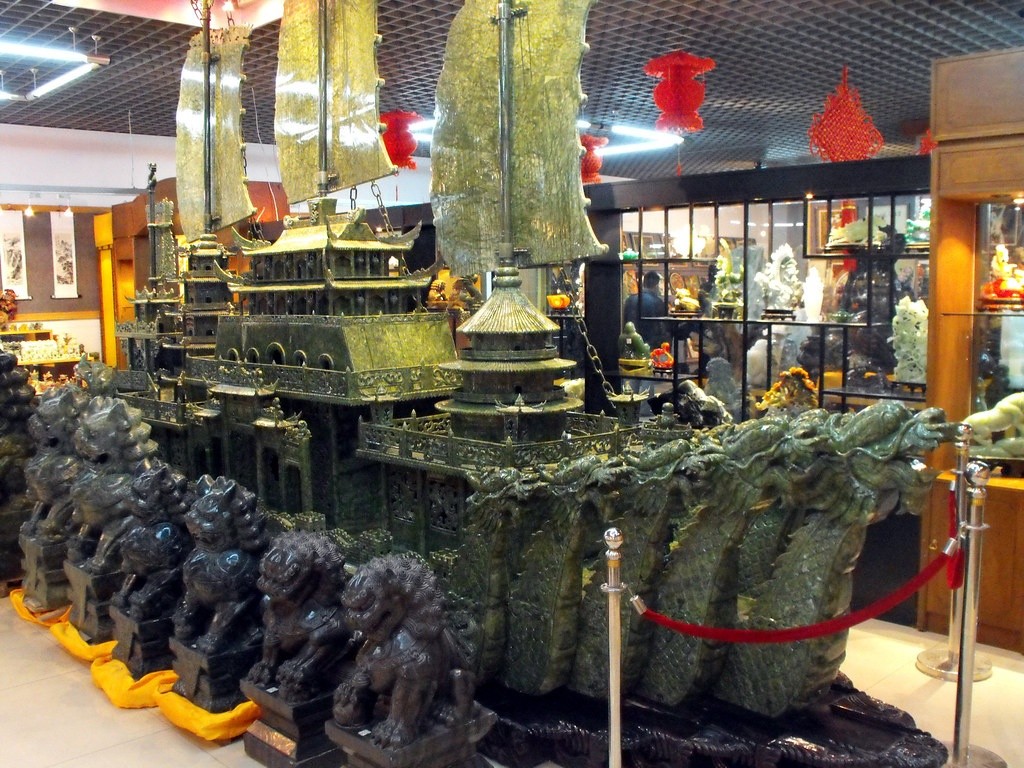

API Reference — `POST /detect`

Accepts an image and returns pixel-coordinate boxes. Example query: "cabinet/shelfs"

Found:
[0,330,95,387]
[112,178,134,371]
[240,151,930,628]
[916,46,1024,655]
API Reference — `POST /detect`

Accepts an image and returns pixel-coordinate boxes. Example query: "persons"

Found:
[624,270,666,419]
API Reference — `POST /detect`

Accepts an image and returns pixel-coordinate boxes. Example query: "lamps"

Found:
[0,26,110,101]
[577,105,684,155]
